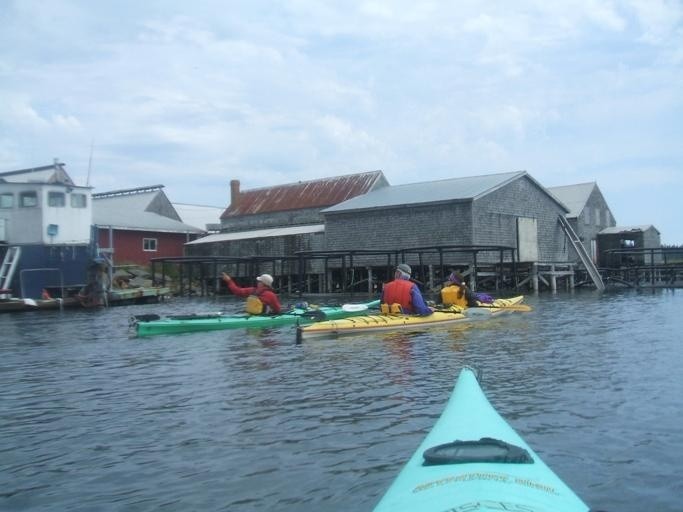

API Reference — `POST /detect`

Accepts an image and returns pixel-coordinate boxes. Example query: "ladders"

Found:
[0,246,20,291]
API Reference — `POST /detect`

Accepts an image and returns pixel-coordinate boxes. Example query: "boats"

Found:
[129,298,382,338]
[373,364,592,508]
[295,295,524,344]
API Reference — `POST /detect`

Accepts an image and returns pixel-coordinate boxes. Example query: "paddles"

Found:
[269,311,326,322]
[466,304,530,312]
[342,303,492,320]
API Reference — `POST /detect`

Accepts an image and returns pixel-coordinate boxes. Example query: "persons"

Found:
[434,269,479,308]
[377,263,439,317]
[220,271,282,316]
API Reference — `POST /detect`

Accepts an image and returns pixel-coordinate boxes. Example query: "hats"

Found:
[390,263,412,279]
[256,273,274,290]
